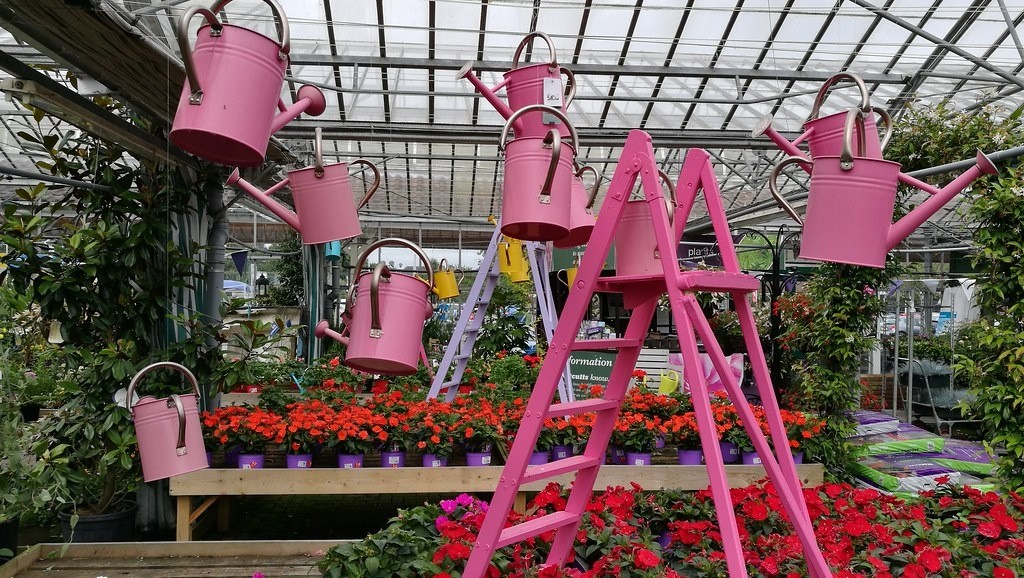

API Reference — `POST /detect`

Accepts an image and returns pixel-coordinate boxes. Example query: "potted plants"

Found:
[921,388,981,419]
[898,359,954,386]
[0,62,307,567]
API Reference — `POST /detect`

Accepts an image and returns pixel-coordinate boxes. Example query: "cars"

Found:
[880,309,941,341]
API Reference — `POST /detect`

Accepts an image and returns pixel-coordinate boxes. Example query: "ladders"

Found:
[427,214,576,425]
[459,128,834,578]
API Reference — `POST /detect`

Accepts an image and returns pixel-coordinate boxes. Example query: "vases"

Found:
[225,444,244,466]
[204,448,213,468]
[626,453,651,465]
[286,453,312,468]
[741,452,761,464]
[466,452,492,466]
[611,445,629,464]
[551,446,572,461]
[237,455,265,470]
[529,451,549,465]
[677,449,703,465]
[338,454,363,468]
[720,443,739,463]
[421,454,447,467]
[381,452,405,467]
[602,452,606,464]
[793,452,804,464]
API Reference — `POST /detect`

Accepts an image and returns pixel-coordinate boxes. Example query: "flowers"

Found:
[306,475,1024,578]
[201,342,826,457]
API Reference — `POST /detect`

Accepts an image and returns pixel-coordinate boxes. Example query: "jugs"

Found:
[754,72,884,174]
[456,32,577,140]
[344,237,433,376]
[770,149,999,268]
[170,0,327,165]
[615,168,678,277]
[498,243,531,282]
[501,105,578,240]
[553,161,600,248]
[656,369,679,396]
[510,257,532,282]
[415,258,464,299]
[126,362,209,483]
[488,215,526,243]
[558,255,608,296]
[226,127,380,244]
[315,285,359,343]
